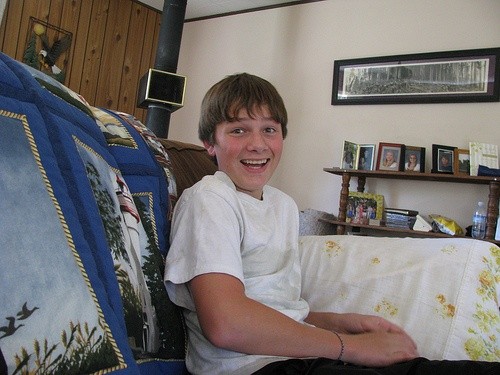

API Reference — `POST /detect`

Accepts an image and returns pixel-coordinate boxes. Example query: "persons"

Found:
[439,155,452,171]
[359,150,371,170]
[164,73,500,375]
[347,201,376,219]
[383,151,397,168]
[344,148,354,168]
[405,154,420,172]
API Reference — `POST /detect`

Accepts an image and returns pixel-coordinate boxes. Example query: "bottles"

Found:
[472,201,486,239]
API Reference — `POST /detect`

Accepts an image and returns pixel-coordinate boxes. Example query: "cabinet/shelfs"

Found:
[318,167,500,246]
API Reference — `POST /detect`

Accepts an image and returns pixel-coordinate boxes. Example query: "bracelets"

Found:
[327,329,344,360]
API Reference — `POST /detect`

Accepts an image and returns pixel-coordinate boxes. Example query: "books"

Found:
[385,208,419,230]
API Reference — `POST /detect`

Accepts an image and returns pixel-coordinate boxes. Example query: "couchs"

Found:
[0,52,500,375]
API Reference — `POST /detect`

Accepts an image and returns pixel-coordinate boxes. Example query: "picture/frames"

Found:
[358,144,376,171]
[454,148,470,176]
[331,47,500,106]
[403,146,425,173]
[346,191,384,225]
[340,140,360,170]
[432,144,458,174]
[376,143,404,172]
